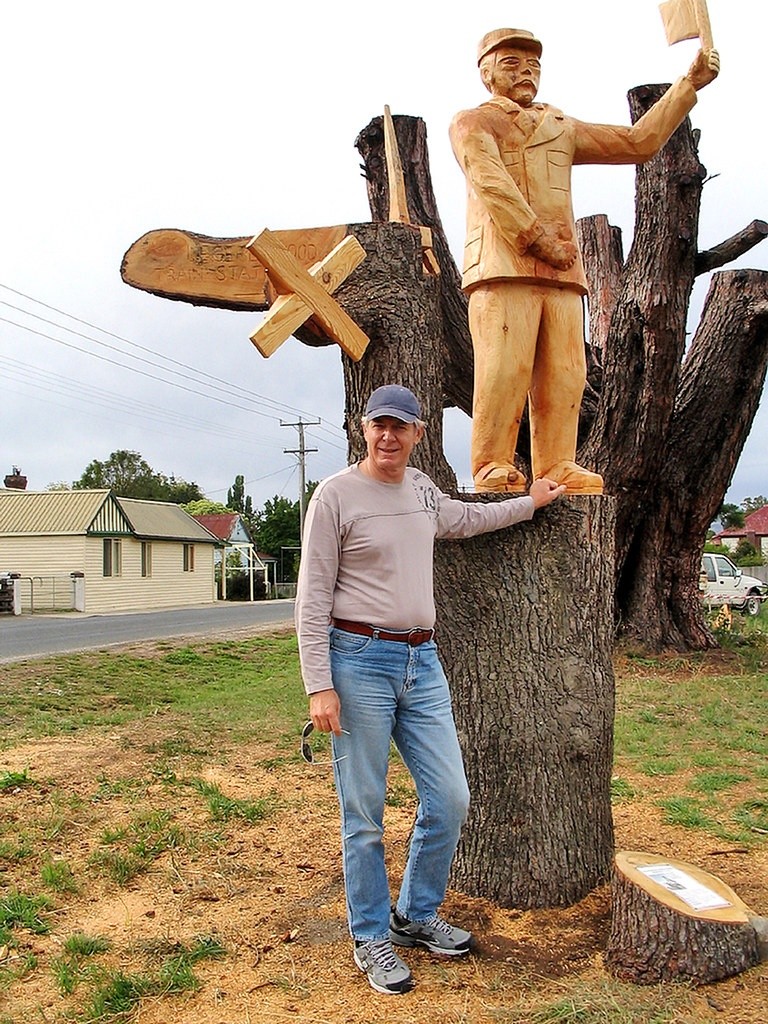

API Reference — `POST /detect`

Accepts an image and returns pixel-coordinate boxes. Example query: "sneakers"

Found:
[352,939,415,995]
[387,906,474,955]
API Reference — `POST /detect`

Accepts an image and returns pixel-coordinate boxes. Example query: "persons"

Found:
[448,28,721,494]
[294,385,569,995]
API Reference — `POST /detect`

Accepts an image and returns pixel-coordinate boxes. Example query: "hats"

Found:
[363,385,423,423]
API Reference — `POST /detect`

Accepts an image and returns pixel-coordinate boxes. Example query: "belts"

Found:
[330,618,432,649]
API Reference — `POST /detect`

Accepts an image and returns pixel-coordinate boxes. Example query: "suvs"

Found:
[697,552,768,615]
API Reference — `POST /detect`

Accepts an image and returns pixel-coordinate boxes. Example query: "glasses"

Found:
[298,720,351,766]
